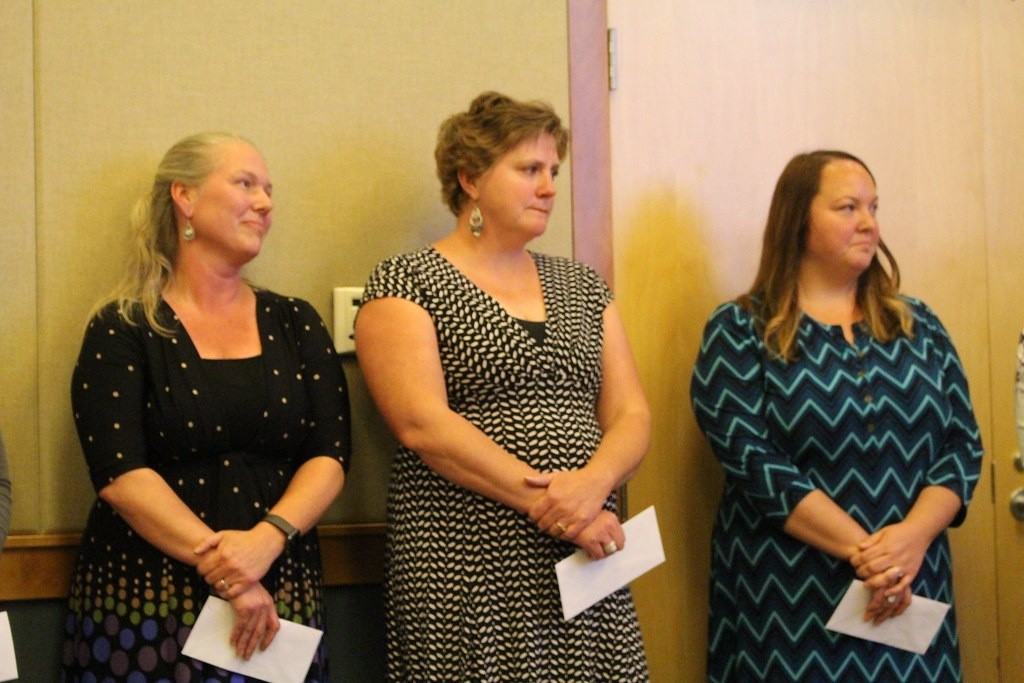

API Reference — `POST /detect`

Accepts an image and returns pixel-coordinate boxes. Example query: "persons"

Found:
[352,92,655,683]
[688,149,983,683]
[0,438,11,548]
[59,131,350,682]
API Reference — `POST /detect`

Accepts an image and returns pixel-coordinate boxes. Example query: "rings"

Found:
[896,573,904,585]
[246,629,252,635]
[886,595,897,603]
[219,580,229,589]
[555,521,566,531]
[605,539,618,554]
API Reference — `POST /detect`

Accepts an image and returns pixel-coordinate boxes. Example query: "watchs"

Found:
[263,515,303,554]
